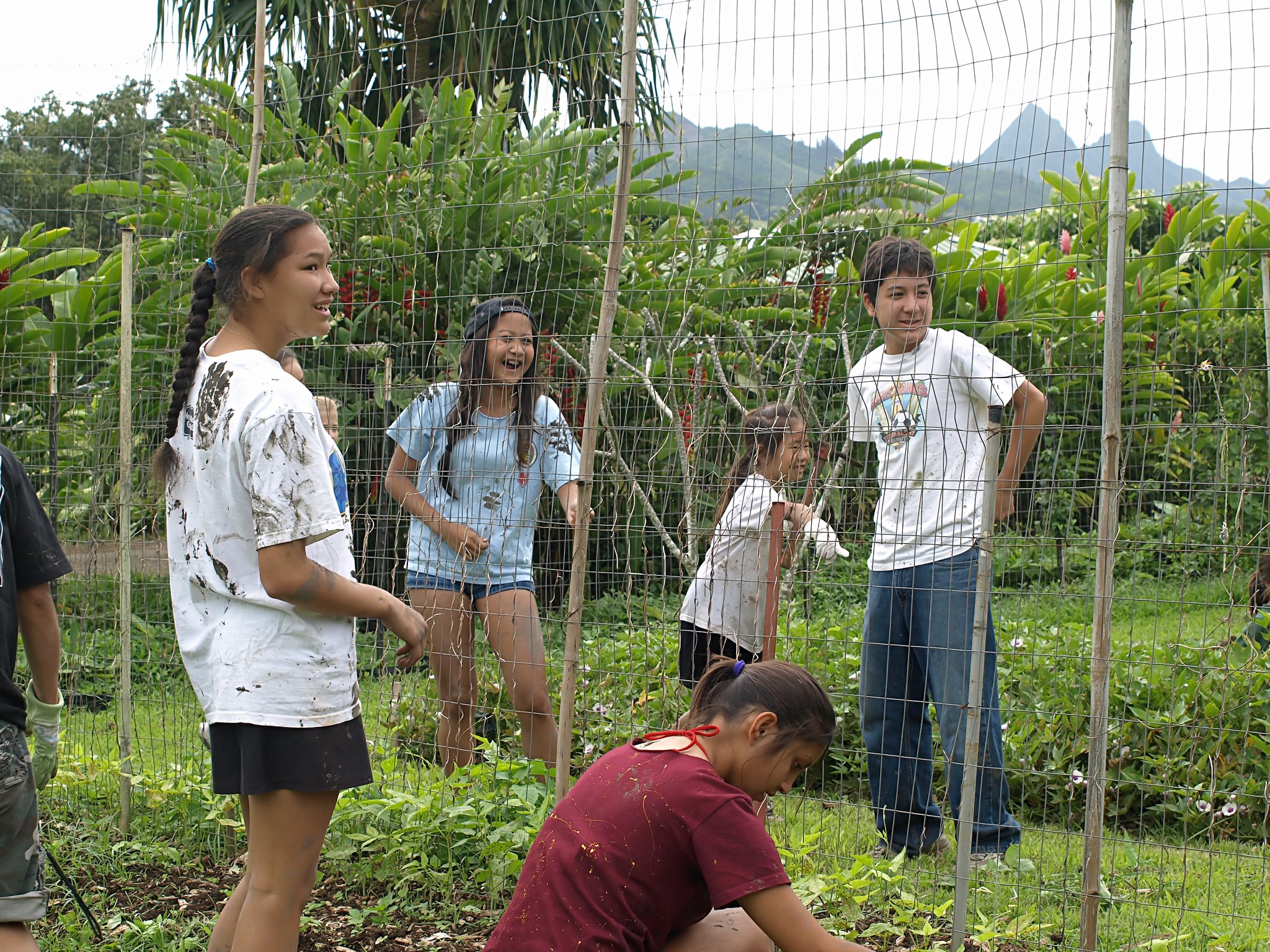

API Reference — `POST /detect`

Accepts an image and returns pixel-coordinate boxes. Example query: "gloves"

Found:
[20,678,66,790]
[805,518,850,567]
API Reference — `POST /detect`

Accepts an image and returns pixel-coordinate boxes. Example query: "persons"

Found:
[483,655,874,951]
[382,297,595,787]
[1233,550,1270,657]
[846,235,1048,870]
[197,346,355,748]
[679,403,851,694]
[156,207,428,952]
[0,442,74,952]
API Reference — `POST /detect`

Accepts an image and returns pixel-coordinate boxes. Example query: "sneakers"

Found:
[862,833,951,870]
[927,850,1007,884]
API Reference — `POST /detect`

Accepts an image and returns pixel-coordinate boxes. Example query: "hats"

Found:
[461,297,538,348]
